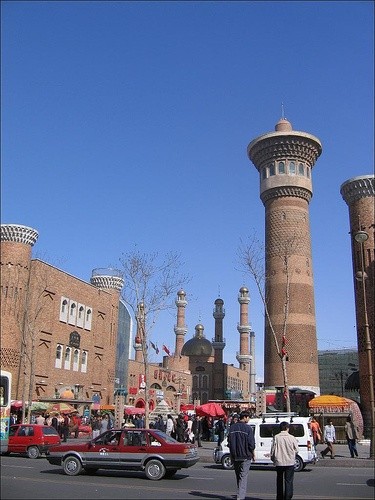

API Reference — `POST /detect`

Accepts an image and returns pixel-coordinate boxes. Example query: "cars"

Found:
[8,424,61,458]
[47,427,200,480]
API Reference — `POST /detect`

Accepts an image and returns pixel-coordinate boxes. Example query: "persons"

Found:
[150,413,203,448]
[201,415,238,447]
[122,413,145,429]
[11,410,80,442]
[90,412,114,439]
[228,411,255,500]
[270,422,299,500]
[310,417,322,450]
[320,419,336,459]
[344,415,358,458]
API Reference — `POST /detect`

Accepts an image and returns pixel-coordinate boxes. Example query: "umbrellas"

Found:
[11,399,50,412]
[43,403,77,415]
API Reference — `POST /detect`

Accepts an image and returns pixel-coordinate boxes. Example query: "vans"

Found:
[214,412,320,473]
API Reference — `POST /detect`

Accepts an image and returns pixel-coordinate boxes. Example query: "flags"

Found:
[149,341,159,354]
[162,345,170,357]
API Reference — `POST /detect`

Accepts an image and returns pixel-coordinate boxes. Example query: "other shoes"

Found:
[330,458,334,459]
[198,445,202,447]
[320,451,324,457]
[356,454,358,456]
[350,456,354,458]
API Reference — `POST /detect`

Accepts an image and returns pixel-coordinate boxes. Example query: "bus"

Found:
[0,370,12,454]
[206,400,256,412]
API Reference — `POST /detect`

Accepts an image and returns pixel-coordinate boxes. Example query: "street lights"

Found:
[274,386,285,413]
[355,214,375,460]
